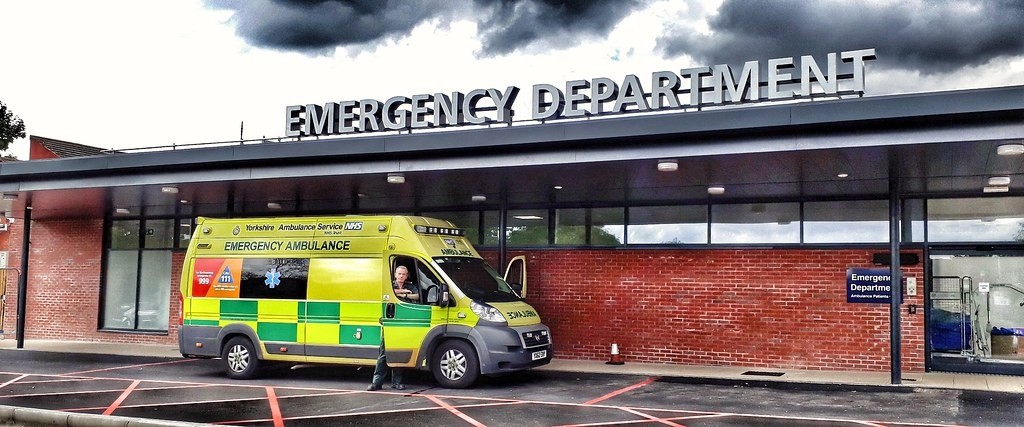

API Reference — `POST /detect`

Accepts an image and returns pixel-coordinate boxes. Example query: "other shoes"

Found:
[367,383,382,391]
[392,383,405,390]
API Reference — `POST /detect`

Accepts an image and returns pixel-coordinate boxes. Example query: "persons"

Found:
[366,266,419,391]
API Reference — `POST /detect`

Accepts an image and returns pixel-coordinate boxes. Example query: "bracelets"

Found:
[404,293,407,299]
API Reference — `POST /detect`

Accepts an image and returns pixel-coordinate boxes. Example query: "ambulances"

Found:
[177,212,554,390]
